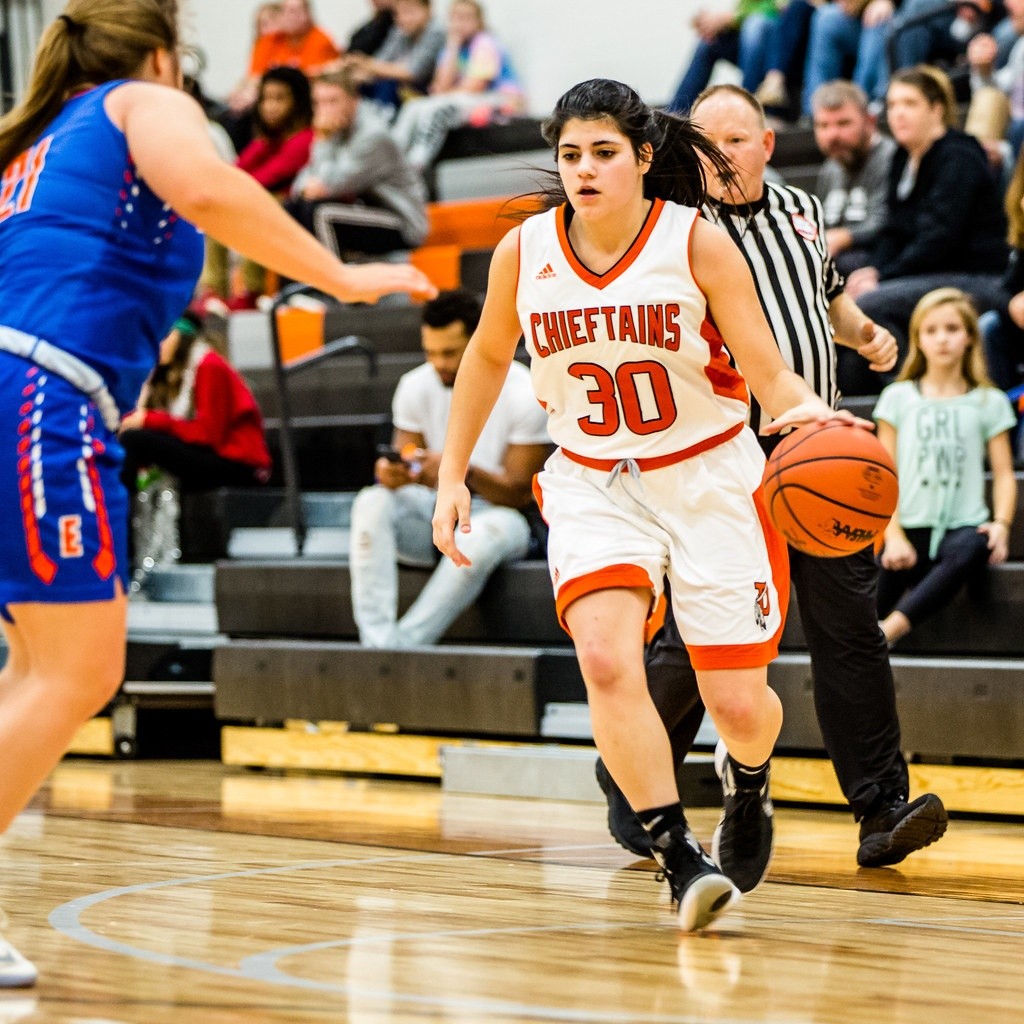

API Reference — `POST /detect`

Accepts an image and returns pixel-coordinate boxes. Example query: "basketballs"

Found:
[762,420,899,558]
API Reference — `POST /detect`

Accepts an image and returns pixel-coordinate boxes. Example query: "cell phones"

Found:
[378,446,403,465]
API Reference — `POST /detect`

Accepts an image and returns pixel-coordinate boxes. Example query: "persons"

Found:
[428,80,876,935]
[597,85,948,872]
[2,0,1024,649]
[1,0,438,995]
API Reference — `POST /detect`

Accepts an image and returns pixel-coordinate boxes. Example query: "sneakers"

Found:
[711,738,774,895]
[596,757,656,860]
[0,938,38,987]
[857,794,949,868]
[642,815,742,931]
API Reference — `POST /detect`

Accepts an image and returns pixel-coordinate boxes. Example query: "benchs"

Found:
[100,94,1024,759]
